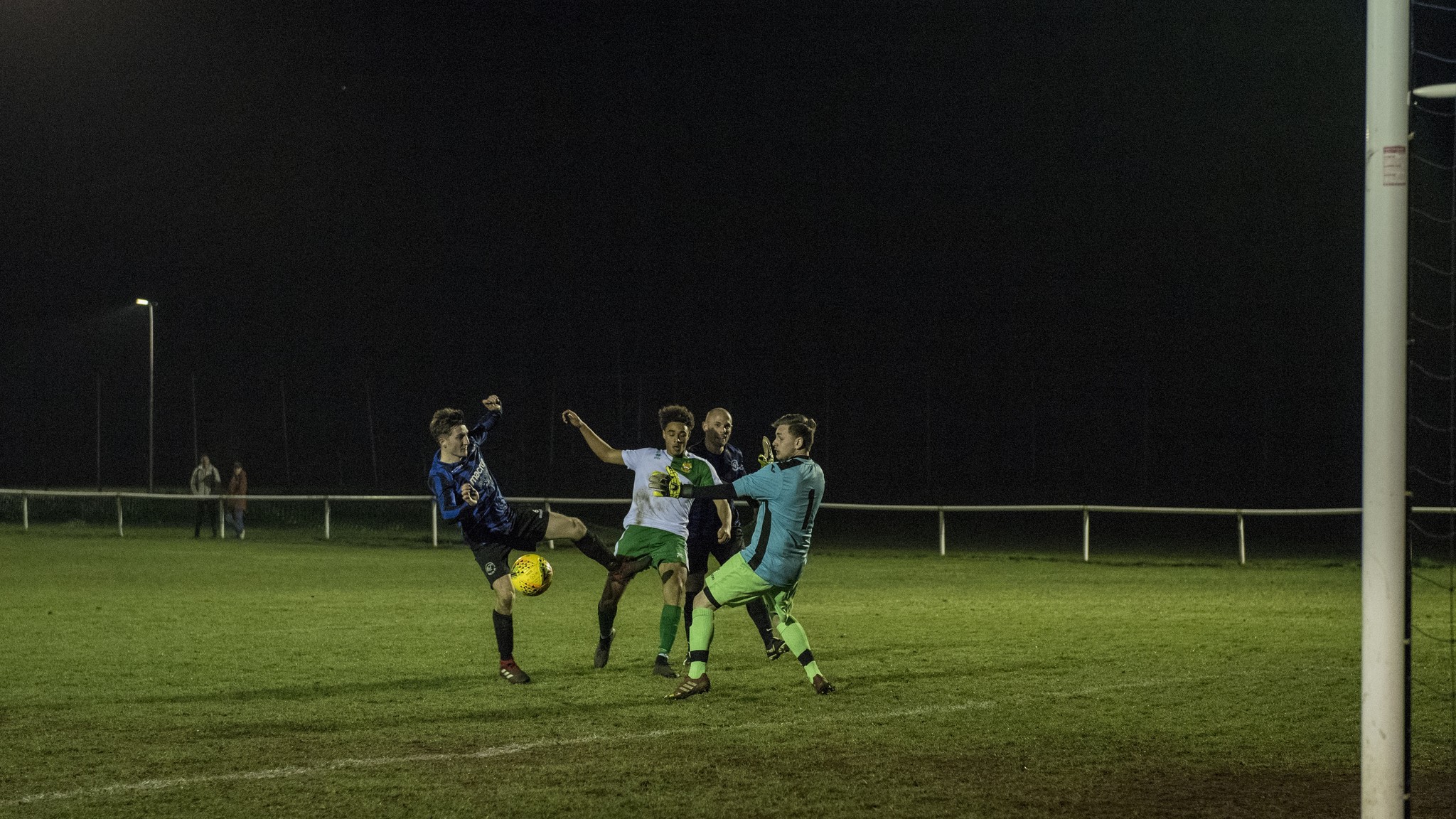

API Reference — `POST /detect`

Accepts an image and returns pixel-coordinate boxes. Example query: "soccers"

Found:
[510,553,554,597]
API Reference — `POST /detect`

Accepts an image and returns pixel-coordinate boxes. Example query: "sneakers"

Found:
[652,654,679,677]
[593,627,616,668]
[765,637,790,661]
[499,658,530,684]
[811,674,833,697]
[664,672,711,701]
[608,555,653,585]
[683,650,692,668]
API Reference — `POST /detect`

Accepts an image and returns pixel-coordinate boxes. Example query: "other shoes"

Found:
[239,528,245,539]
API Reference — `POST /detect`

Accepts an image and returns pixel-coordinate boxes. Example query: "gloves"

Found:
[758,435,774,469]
[648,466,694,499]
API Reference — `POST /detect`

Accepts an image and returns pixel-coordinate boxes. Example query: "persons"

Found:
[561,403,732,678]
[427,394,652,684]
[681,408,789,665]
[191,454,221,540]
[224,461,248,539]
[648,413,835,700]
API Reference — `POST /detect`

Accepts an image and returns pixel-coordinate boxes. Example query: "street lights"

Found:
[135,298,154,492]
